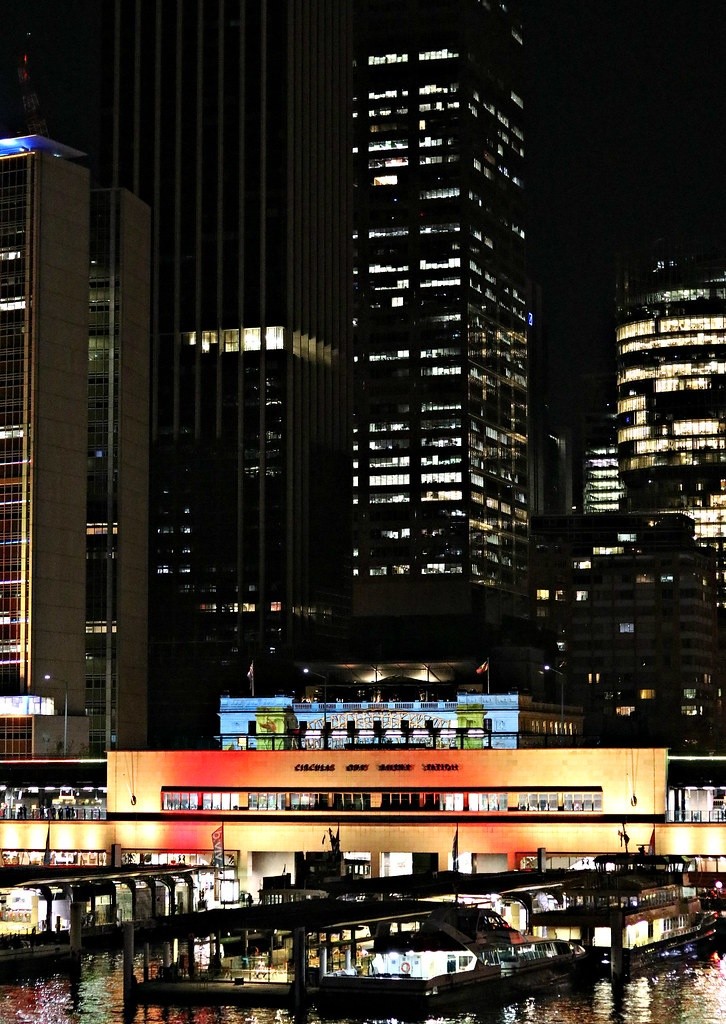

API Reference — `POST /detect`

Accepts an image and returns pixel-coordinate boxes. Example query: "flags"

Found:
[477,662,489,674]
[246,663,254,681]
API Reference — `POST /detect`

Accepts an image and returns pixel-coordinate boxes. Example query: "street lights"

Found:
[544,665,565,736]
[302,668,328,727]
[44,673,68,759]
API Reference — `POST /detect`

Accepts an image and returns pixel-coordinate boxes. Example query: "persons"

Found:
[0,802,75,819]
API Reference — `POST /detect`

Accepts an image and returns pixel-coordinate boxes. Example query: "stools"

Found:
[373,721,387,749]
[322,722,333,749]
[347,721,360,749]
[299,721,307,749]
[401,720,414,750]
[456,728,469,749]
[425,720,442,749]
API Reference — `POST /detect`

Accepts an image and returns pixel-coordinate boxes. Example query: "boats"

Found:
[0,927,71,964]
[524,851,726,968]
[313,902,592,1009]
